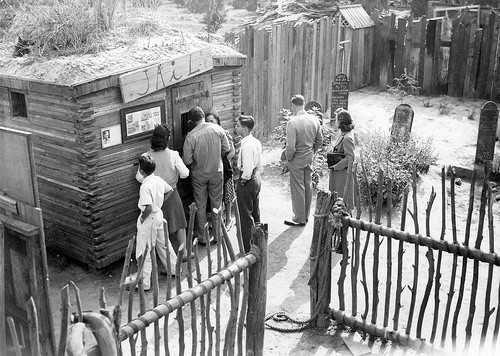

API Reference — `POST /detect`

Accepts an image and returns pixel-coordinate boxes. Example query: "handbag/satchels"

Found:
[327,152,348,169]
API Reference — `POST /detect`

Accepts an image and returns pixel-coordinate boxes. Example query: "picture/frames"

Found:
[119,100,167,144]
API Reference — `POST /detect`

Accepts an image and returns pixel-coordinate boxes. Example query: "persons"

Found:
[235,115,262,253]
[285,95,323,226]
[136,125,194,261]
[184,107,235,246]
[126,156,182,291]
[329,108,355,216]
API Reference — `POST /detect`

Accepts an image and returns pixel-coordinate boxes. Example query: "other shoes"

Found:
[127,286,149,292]
[161,270,175,277]
[182,252,194,261]
[197,241,207,246]
[285,219,305,226]
